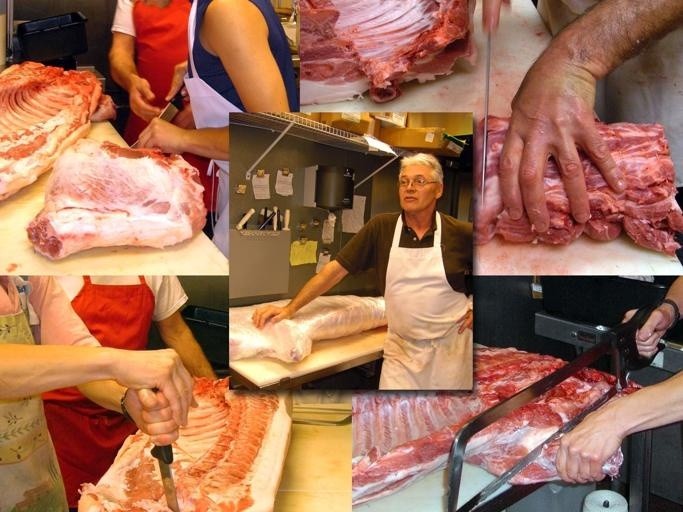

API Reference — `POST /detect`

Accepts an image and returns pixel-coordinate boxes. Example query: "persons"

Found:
[107,0,221,241]
[26,276,218,512]
[138,0,299,261]
[250,153,472,389]
[554,276,682,485]
[0,276,198,511]
[481,0,683,262]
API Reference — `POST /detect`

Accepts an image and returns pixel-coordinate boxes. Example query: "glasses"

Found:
[395,178,438,189]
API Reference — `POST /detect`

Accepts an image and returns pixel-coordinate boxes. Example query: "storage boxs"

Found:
[290,112,465,157]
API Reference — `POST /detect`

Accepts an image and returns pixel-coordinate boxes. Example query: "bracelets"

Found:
[661,298,681,330]
[119,387,136,425]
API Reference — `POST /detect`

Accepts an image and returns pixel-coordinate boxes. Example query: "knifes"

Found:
[151,446,180,512]
[130,95,183,147]
[235,206,292,230]
